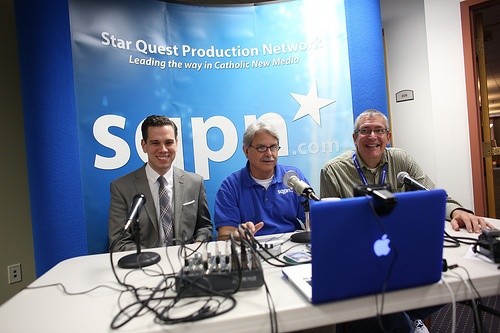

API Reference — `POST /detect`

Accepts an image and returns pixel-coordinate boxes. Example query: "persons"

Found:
[109,115,213,253]
[214,121,311,241]
[320,109,496,333]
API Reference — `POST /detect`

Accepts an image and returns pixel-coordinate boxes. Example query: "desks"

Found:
[0,216,500,333]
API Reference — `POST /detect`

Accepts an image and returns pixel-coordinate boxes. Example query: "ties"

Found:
[156,176,175,247]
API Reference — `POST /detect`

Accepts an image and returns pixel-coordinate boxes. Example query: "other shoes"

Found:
[413,319,430,333]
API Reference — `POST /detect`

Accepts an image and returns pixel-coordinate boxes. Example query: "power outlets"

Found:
[7,263,22,285]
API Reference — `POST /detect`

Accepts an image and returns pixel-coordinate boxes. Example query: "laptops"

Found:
[280,189,448,305]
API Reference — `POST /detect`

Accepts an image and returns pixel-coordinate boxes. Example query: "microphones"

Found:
[122,193,146,236]
[288,176,320,202]
[397,172,427,191]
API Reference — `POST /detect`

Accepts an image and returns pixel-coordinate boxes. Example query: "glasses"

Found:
[355,127,390,135]
[249,144,281,153]
